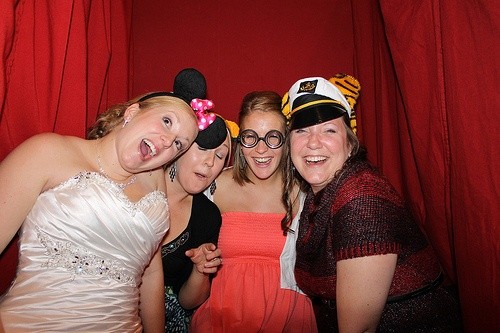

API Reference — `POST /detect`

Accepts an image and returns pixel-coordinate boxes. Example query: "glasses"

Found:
[237,129,286,149]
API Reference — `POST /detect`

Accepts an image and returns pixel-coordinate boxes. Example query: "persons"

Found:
[188,90,323,333]
[282,73,464,333]
[0,90,199,333]
[162,114,233,333]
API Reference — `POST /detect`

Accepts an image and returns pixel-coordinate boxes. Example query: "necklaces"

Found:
[96,137,138,192]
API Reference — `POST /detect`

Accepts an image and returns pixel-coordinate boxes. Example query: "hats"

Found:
[285,77,352,132]
